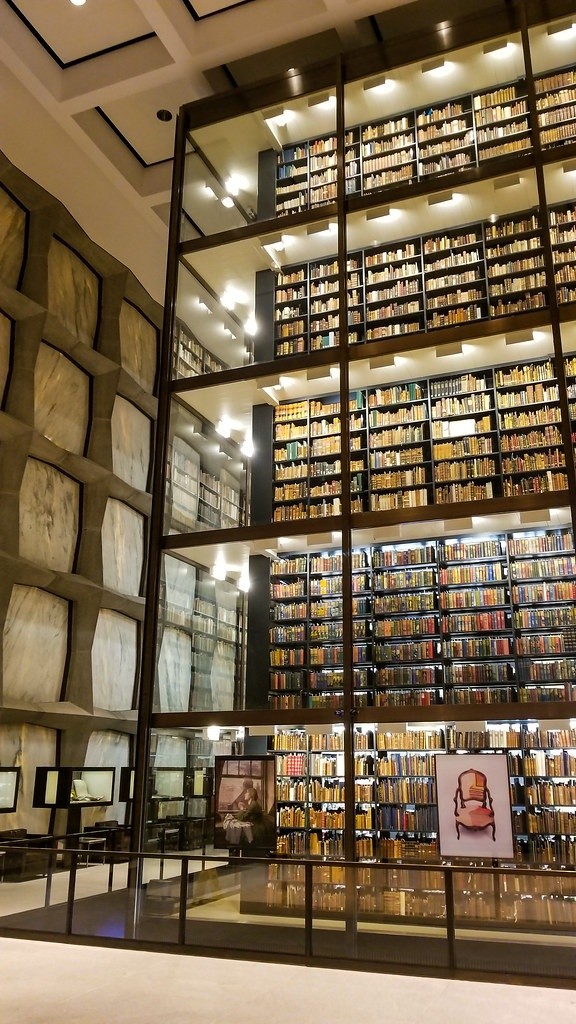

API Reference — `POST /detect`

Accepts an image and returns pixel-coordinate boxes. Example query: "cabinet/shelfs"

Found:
[249,0,576,931]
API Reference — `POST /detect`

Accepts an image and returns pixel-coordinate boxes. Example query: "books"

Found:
[273,355,576,522]
[274,729,576,869]
[276,69,576,356]
[270,532,576,709]
